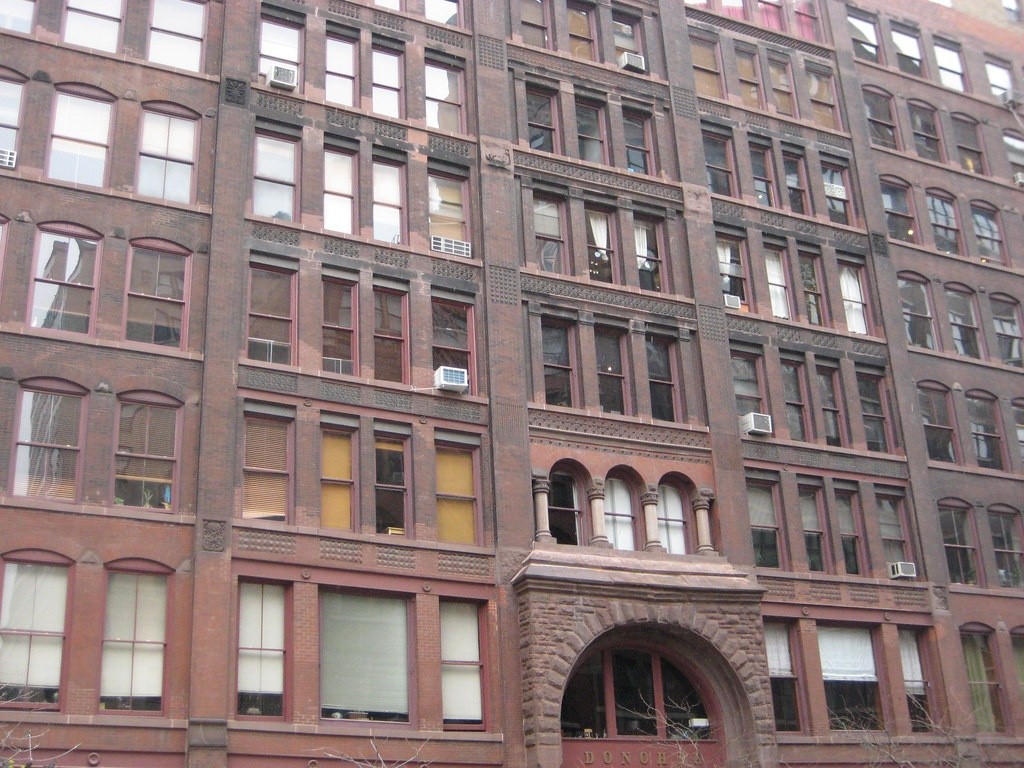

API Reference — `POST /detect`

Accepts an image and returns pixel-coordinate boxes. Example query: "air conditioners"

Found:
[617,50,646,74]
[740,412,774,435]
[432,365,469,393]
[263,62,296,90]
[722,292,742,311]
[1013,172,1024,186]
[889,560,918,580]
[431,235,472,259]
